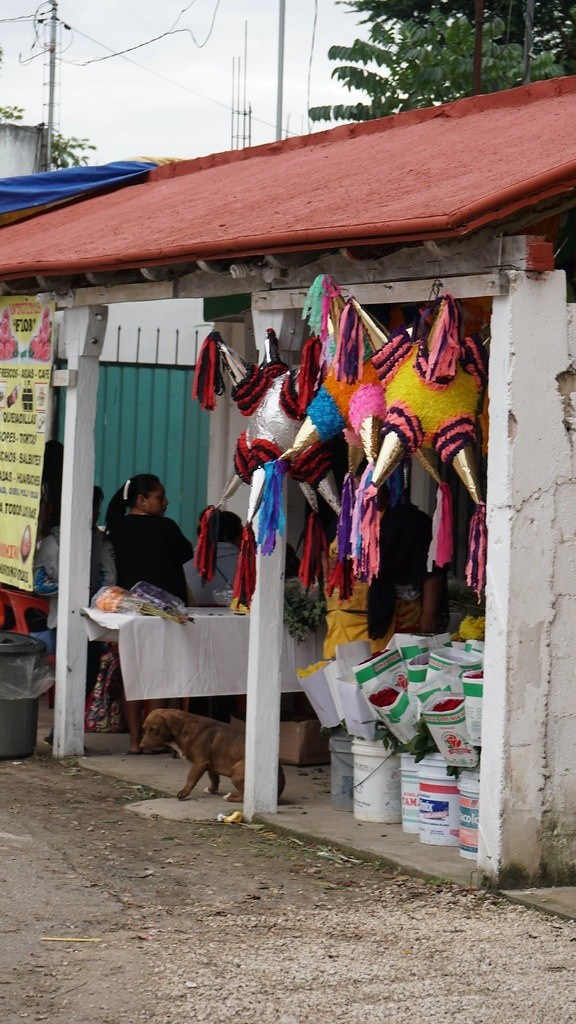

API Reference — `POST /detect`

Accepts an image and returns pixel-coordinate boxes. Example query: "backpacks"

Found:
[83,640,129,735]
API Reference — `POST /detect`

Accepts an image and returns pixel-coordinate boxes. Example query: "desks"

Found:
[78,606,323,759]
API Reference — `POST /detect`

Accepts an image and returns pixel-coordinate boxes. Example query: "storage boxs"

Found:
[228,712,329,767]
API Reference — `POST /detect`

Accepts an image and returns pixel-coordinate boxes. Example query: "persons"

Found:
[397,490,450,637]
[323,485,397,658]
[34,485,116,750]
[183,511,248,607]
[103,474,194,754]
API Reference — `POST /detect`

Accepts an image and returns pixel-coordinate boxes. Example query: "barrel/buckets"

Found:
[457,770,479,861]
[350,739,402,824]
[328,734,354,812]
[399,751,420,834]
[418,752,460,847]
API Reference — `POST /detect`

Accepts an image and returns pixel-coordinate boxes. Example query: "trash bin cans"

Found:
[0,629,48,760]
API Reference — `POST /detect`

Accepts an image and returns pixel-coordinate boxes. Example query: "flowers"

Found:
[297,610,484,768]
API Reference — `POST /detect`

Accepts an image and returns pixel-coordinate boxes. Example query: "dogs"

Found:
[139,708,286,803]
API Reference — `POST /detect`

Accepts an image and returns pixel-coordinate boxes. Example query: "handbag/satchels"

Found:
[32,528,58,596]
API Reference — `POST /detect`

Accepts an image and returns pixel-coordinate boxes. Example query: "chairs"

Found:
[0,587,55,709]
